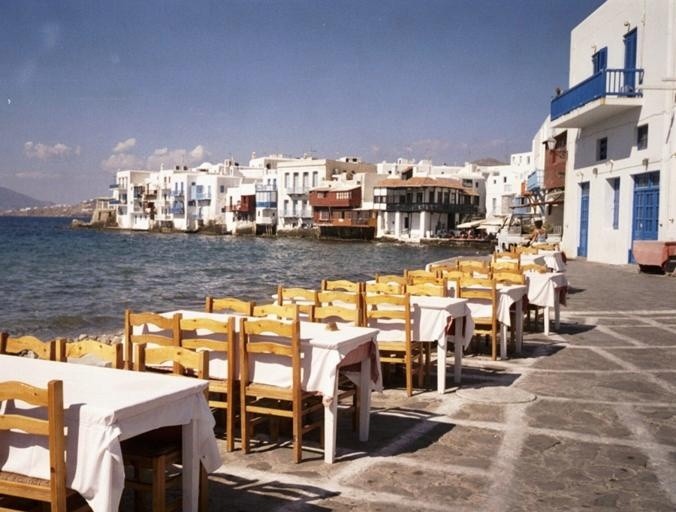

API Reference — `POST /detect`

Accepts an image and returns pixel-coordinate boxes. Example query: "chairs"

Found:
[120,297,384,463]
[273,233,576,396]
[2,333,209,511]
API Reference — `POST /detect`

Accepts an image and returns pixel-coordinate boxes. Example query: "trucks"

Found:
[497,213,562,253]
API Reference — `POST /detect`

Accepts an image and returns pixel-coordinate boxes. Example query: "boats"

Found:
[420,231,493,248]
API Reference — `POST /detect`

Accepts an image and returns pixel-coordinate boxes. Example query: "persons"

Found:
[444,230,491,239]
[530,221,547,245]
[554,87,563,96]
